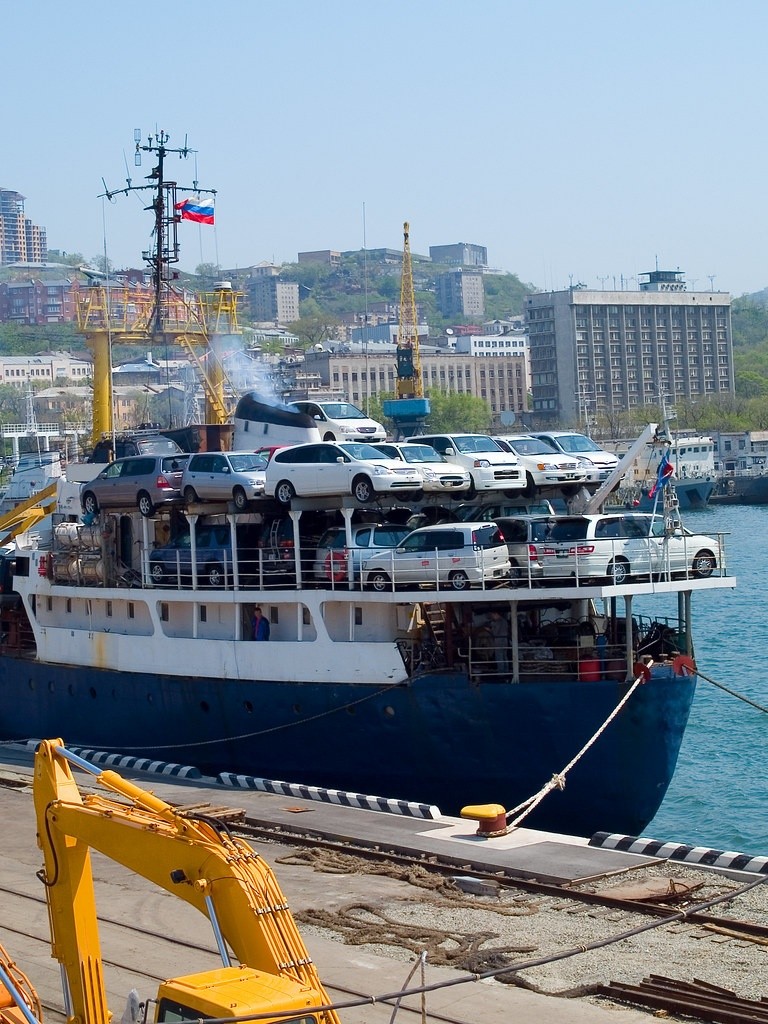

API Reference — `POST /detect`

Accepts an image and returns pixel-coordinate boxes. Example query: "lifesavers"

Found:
[325,554,347,581]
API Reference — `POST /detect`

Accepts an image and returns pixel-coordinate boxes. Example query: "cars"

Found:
[364,442,471,502]
[528,432,627,493]
[300,499,559,527]
[491,435,588,498]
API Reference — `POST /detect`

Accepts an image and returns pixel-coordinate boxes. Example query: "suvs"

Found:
[80,453,191,518]
[180,451,284,511]
[286,399,387,443]
[91,432,185,464]
[490,512,568,586]
[149,522,263,586]
[542,512,725,586]
[313,521,418,592]
[264,441,423,506]
[360,522,512,591]
[256,516,342,581]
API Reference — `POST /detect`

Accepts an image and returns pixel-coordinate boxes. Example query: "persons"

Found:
[252,607,270,641]
[492,609,508,677]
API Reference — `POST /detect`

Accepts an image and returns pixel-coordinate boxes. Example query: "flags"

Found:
[175,197,214,224]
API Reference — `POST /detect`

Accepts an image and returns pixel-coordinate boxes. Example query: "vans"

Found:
[403,433,528,501]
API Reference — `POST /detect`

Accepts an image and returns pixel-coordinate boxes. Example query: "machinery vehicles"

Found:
[1,738,341,1024]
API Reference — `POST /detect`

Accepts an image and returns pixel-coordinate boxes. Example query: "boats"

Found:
[595,381,719,512]
[708,479,747,504]
[0,422,737,839]
